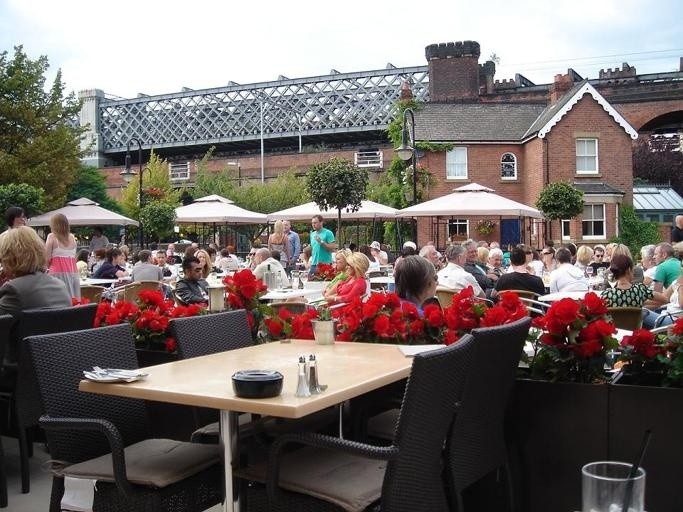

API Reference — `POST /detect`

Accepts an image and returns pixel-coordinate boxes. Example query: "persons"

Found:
[302,215,339,279]
[671,215,683,256]
[77,239,683,344]
[0,225,73,395]
[6,208,82,300]
[269,220,300,277]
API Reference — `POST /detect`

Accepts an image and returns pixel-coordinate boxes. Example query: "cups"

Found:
[291,270,299,291]
[210,271,216,280]
[596,267,605,275]
[387,264,393,278]
[581,459,646,511]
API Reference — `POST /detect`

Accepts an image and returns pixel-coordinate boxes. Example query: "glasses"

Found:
[248,252,256,256]
[523,251,532,255]
[595,254,604,258]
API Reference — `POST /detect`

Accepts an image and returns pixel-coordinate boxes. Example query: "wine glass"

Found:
[585,266,594,281]
[299,271,308,291]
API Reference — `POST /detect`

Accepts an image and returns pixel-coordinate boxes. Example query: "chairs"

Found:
[0,255,682,511]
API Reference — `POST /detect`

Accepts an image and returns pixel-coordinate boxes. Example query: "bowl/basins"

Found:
[229,369,283,399]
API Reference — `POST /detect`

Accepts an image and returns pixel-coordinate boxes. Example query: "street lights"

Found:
[227,162,241,189]
[118,138,152,250]
[394,108,417,257]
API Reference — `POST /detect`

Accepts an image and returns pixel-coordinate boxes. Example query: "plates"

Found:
[83,368,128,383]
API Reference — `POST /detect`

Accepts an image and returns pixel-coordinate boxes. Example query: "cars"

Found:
[251,224,275,252]
[182,224,251,252]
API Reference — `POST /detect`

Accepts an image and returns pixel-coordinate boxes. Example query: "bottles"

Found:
[263,264,275,292]
[80,268,87,282]
[276,271,283,289]
[294,355,311,398]
[307,354,322,396]
[225,266,229,275]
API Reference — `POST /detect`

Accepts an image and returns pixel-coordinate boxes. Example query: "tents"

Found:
[24,197,139,246]
[174,193,399,248]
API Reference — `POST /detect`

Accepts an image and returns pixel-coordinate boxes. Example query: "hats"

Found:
[368,240,381,251]
[402,240,417,250]
